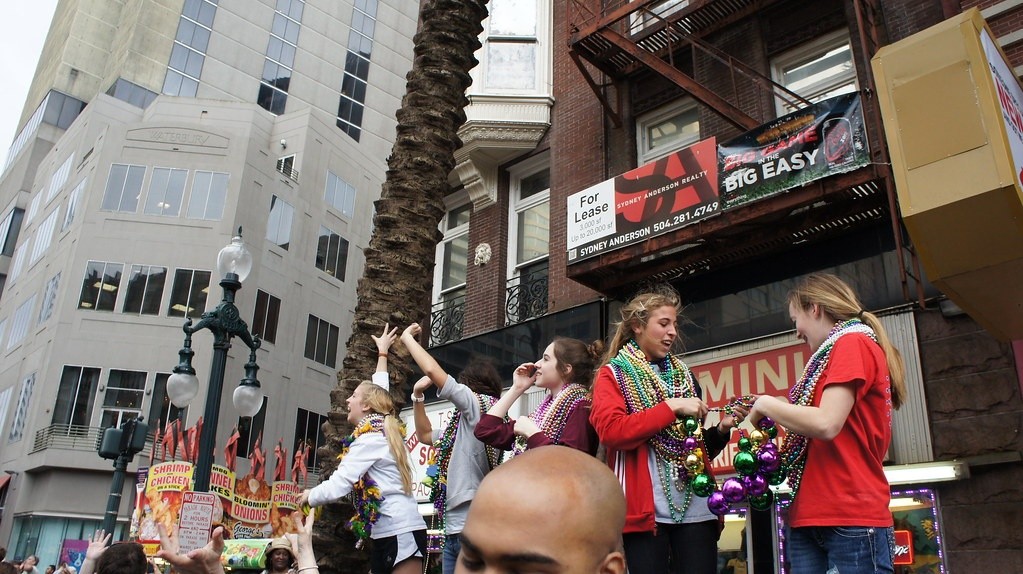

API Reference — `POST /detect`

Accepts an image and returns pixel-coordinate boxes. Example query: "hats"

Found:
[265,537,295,559]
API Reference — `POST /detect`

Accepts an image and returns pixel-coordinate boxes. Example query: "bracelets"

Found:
[378,353,388,357]
[411,393,425,402]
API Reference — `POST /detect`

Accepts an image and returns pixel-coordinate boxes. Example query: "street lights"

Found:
[165,229,265,496]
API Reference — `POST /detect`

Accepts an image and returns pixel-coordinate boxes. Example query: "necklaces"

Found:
[775,318,879,509]
[337,414,408,549]
[509,382,593,458]
[424,394,508,574]
[609,339,788,522]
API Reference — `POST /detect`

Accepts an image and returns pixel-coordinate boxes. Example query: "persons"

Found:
[401,323,508,574]
[474,337,604,458]
[0,529,162,574]
[295,323,428,574]
[748,274,905,574]
[261,539,297,574]
[157,508,319,574]
[589,291,748,574]
[454,445,627,574]
